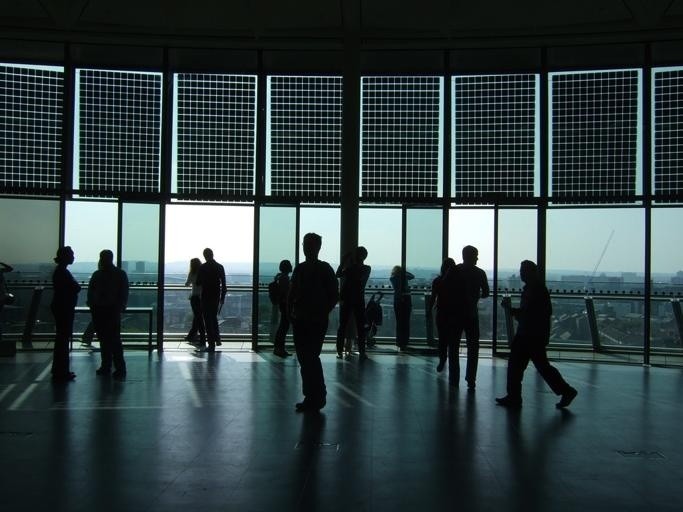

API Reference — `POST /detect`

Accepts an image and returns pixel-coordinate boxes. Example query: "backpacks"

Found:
[267,273,287,307]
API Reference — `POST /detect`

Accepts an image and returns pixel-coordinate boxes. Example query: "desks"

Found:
[67,305,153,350]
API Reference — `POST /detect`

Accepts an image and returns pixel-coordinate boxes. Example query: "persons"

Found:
[495,260,578,408]
[390,266,415,350]
[81,259,102,346]
[440,245,489,388]
[335,246,371,361]
[85,250,129,378]
[185,258,206,352]
[50,246,81,380]
[196,248,227,352]
[425,258,456,372]
[286,233,339,410]
[268,260,292,358]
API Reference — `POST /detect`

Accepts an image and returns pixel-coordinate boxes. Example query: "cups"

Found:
[502,294,512,299]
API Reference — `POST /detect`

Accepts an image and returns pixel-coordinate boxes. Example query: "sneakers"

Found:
[48,366,126,386]
[554,388,579,409]
[295,392,327,413]
[271,348,295,360]
[494,389,524,407]
[396,346,416,354]
[186,335,223,352]
[334,351,370,362]
[434,363,477,389]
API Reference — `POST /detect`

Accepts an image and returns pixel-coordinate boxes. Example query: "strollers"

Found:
[364,290,386,348]
